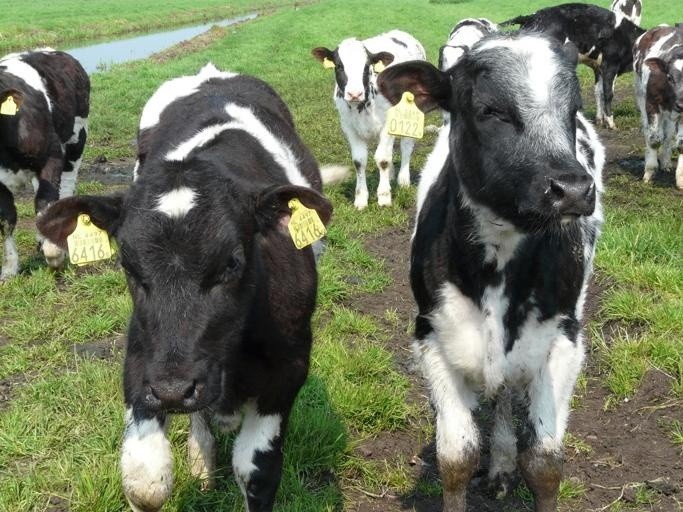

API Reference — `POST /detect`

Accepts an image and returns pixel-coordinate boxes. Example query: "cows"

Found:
[510,1,646,130]
[375,21,607,512]
[0,46,92,279]
[631,23,683,194]
[34,58,351,512]
[308,27,427,211]
